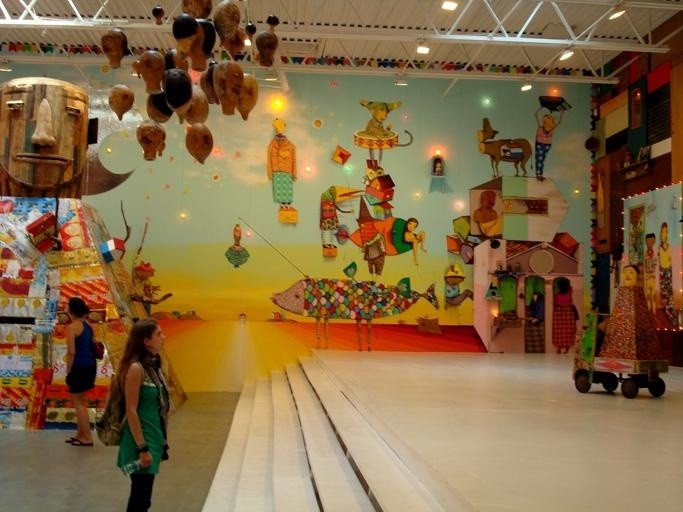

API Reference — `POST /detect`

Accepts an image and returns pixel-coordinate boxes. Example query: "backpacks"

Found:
[96,372,127,446]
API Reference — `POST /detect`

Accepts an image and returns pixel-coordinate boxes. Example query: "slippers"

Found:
[65,437,93,446]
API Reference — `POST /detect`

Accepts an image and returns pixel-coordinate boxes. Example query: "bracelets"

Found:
[139,445,149,455]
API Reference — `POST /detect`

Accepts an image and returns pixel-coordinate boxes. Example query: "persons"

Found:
[114,318,170,512]
[64,294,94,447]
[656,221,678,332]
[644,232,657,317]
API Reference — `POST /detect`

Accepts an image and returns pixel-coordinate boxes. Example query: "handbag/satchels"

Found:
[93,341,104,359]
